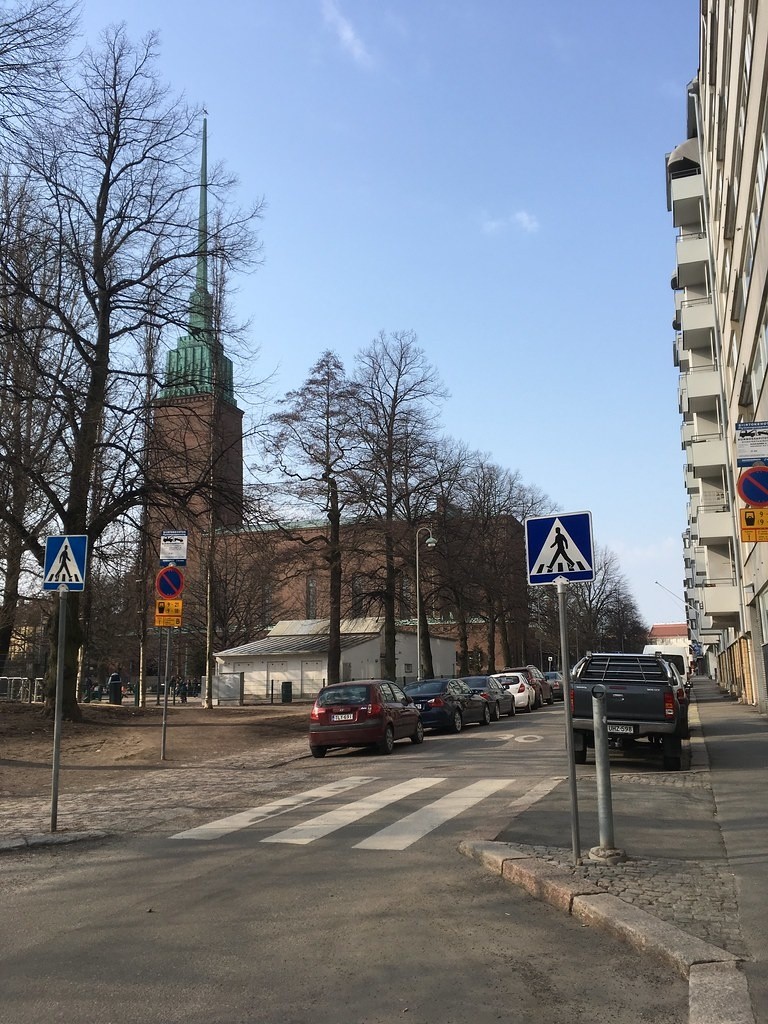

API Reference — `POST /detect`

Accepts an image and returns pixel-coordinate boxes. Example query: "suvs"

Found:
[569,645,693,771]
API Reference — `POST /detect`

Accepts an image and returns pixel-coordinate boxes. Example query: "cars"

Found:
[309,680,424,758]
[403,678,490,733]
[459,676,515,721]
[490,665,579,713]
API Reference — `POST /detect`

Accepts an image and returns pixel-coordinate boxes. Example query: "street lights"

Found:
[416,527,437,681]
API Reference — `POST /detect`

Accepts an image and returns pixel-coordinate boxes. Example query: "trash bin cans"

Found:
[282,681,292,703]
[109,681,122,704]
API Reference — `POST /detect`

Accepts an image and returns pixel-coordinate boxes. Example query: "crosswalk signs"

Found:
[43,536,87,592]
[528,514,595,586]
